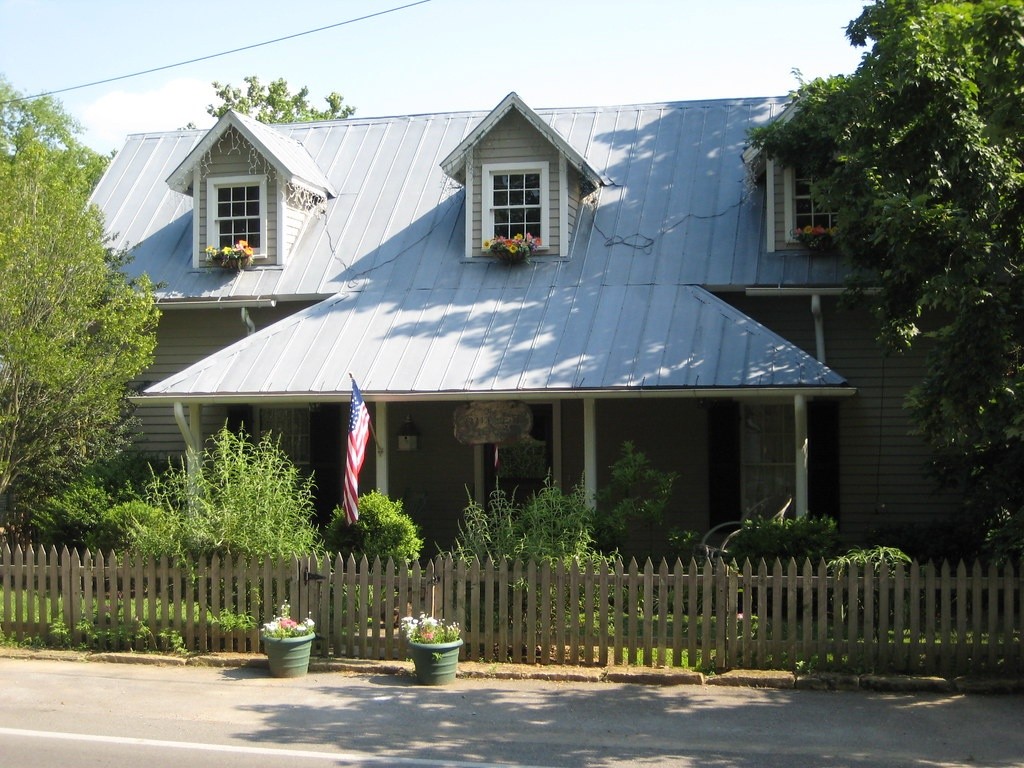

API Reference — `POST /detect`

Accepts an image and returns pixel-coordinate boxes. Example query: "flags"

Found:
[339,384,371,527]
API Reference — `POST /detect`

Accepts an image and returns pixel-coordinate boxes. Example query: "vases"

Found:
[263,632,315,677]
[409,637,463,686]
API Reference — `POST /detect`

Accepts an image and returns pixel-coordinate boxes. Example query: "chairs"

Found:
[401,487,427,521]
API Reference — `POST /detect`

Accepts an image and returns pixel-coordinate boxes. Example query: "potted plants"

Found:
[727,511,840,610]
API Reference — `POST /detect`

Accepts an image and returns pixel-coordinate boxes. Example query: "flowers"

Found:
[401,612,461,644]
[260,599,315,637]
[790,225,838,251]
[206,240,254,274]
[483,232,542,264]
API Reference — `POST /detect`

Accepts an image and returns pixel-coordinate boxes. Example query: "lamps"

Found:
[396,414,418,451]
